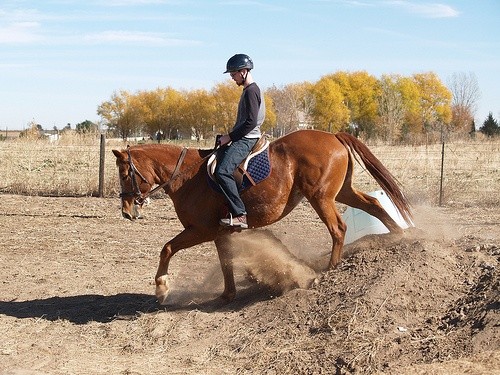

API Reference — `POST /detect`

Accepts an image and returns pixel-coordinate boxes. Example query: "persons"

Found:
[207,54,265,229]
[156,131,163,144]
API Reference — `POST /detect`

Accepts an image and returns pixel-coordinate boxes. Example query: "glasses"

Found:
[230,71,240,77]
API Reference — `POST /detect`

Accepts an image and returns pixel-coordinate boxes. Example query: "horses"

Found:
[112,129,416,310]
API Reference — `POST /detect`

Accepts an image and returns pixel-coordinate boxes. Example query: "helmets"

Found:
[223,54,253,74]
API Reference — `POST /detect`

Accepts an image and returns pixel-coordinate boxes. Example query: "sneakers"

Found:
[219,214,248,228]
